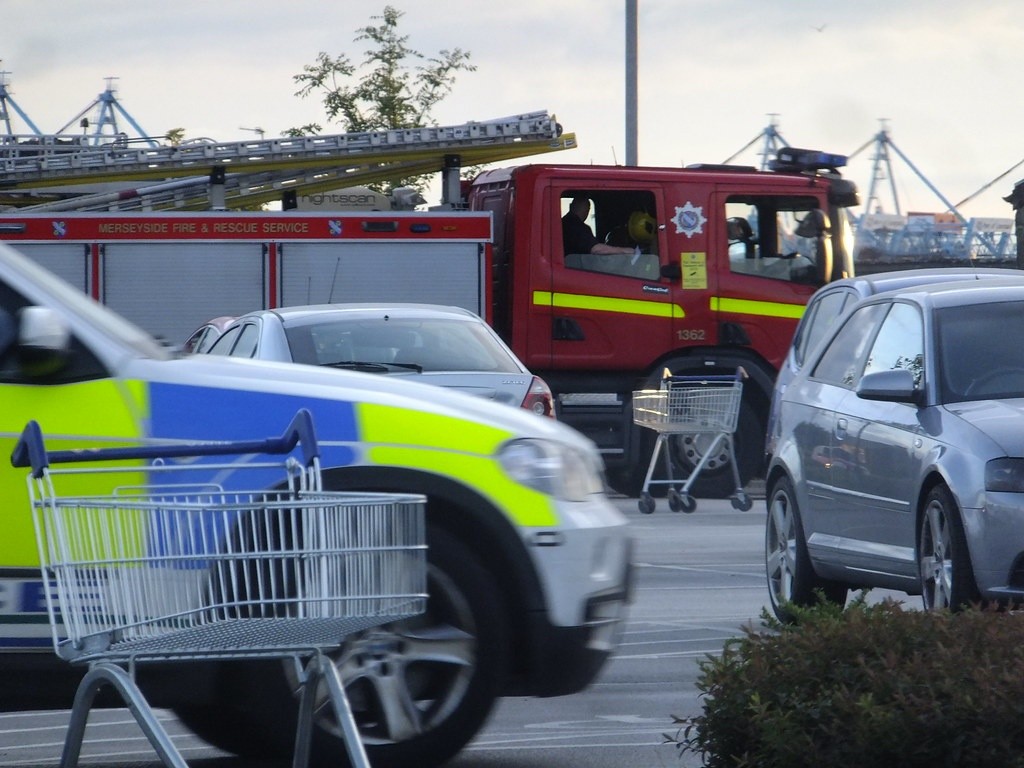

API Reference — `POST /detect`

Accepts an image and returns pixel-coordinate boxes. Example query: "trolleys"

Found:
[633,366,755,513]
[10,409,430,768]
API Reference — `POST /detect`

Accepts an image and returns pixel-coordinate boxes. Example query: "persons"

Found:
[562,196,638,255]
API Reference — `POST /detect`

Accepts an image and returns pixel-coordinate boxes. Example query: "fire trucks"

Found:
[0,67,866,496]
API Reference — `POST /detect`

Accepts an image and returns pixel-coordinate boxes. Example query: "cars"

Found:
[173,316,239,357]
[766,278,1024,623]
[209,300,558,419]
[763,266,1024,476]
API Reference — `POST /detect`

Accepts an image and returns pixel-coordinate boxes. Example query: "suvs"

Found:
[0,246,643,767]
